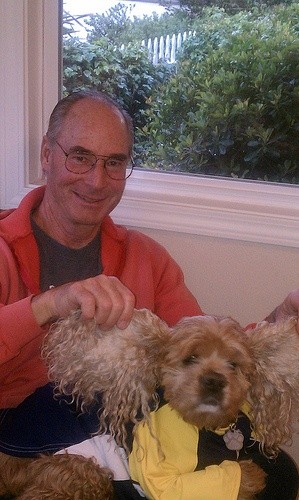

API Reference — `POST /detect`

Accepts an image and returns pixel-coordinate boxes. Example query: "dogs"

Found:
[0,306,299,500]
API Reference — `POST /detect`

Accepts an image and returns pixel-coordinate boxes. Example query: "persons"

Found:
[0,90,299,500]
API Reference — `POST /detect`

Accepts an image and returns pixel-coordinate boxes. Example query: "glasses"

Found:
[52,137,135,181]
